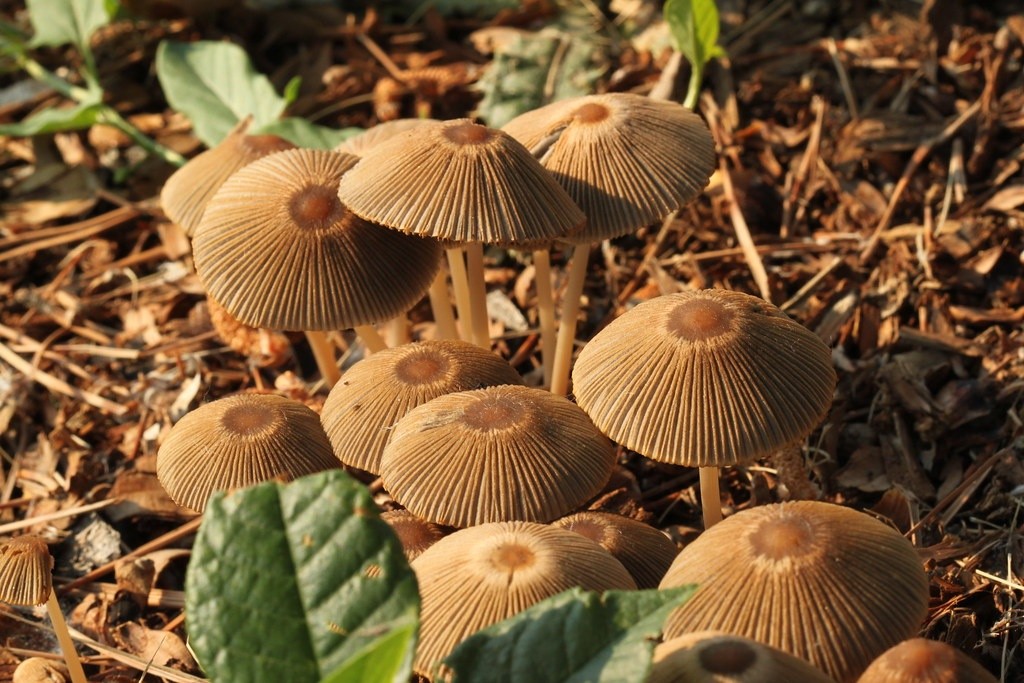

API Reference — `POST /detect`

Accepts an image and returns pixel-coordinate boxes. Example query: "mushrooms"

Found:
[0,91,1003,683]
